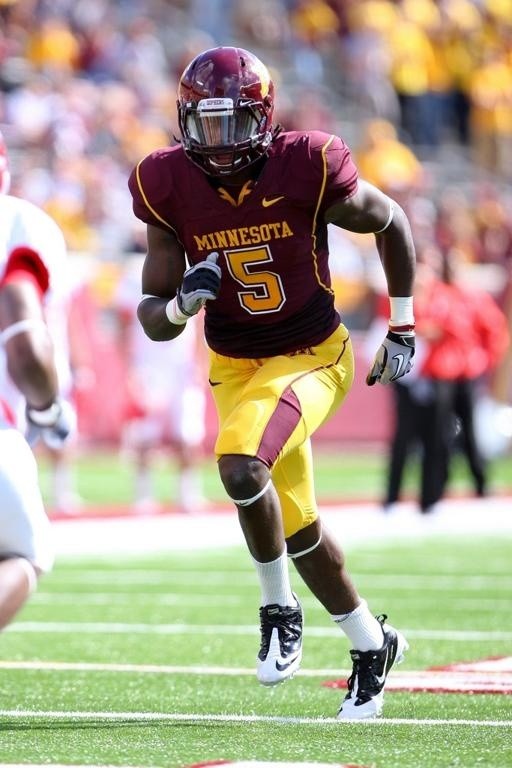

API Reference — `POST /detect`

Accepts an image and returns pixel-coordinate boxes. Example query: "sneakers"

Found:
[337,623,409,722]
[257,591,304,688]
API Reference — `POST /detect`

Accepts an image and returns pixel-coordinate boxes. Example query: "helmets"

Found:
[176,46,275,178]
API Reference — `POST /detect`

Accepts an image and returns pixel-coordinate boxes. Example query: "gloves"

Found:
[25,402,70,448]
[366,296,417,387]
[166,251,224,325]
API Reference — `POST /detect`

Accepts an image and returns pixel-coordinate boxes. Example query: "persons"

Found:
[0,125,81,633]
[124,46,419,724]
[0,0,512,518]
